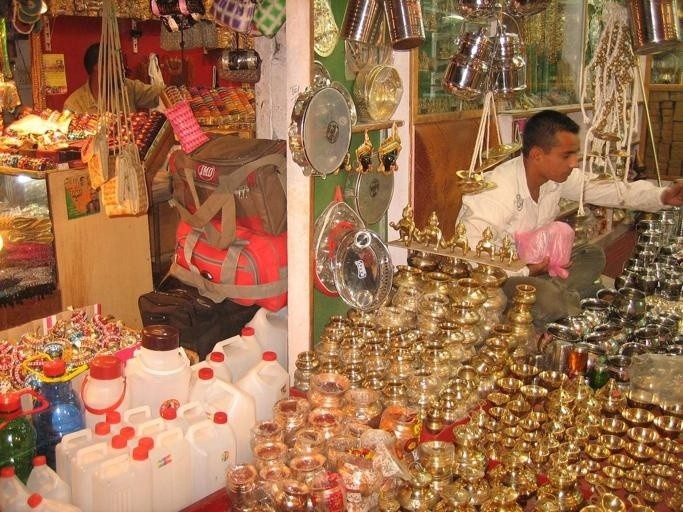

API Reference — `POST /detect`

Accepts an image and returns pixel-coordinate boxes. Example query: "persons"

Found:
[61,41,166,124]
[452,111,682,280]
[78,177,87,185]
[86,189,100,215]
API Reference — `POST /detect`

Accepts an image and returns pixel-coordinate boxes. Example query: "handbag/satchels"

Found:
[175,208,289,312]
[167,134,288,236]
[81,134,150,216]
[150,0,286,39]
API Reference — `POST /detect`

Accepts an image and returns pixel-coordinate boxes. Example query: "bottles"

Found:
[0,385,51,484]
[78,354,131,432]
[123,323,191,422]
[30,358,86,473]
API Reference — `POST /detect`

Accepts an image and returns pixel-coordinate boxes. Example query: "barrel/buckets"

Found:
[189,302,289,468]
[1,455,83,511]
[56,398,236,512]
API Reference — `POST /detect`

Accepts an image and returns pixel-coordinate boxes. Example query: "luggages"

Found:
[137,275,256,357]
